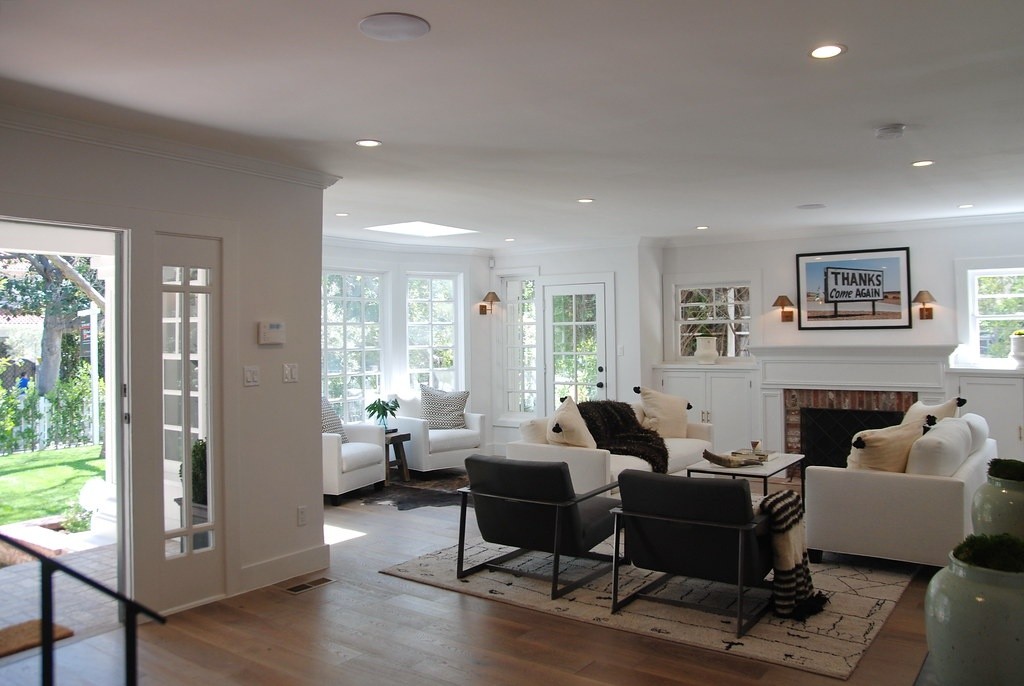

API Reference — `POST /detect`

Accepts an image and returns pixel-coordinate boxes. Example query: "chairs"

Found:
[453,453,800,639]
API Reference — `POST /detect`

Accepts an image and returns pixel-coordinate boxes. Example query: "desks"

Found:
[667,449,805,523]
[385,433,412,485]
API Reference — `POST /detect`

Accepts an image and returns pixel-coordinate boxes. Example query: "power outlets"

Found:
[298,506,306,525]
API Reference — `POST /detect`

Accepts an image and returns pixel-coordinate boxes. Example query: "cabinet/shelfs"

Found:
[650,365,756,453]
[945,368,1024,459]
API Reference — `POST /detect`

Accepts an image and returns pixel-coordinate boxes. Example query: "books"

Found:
[731,448,777,461]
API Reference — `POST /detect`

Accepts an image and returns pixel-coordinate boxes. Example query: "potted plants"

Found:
[924,458,1024,686]
[1009,330,1024,362]
[173,436,209,551]
[694,332,718,365]
[365,397,400,433]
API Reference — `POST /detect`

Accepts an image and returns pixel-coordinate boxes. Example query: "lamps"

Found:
[479,292,500,315]
[772,295,794,323]
[912,291,937,319]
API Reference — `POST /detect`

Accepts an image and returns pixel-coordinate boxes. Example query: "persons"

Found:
[17,371,29,399]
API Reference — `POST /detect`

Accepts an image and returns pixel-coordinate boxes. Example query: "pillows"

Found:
[635,384,692,437]
[421,384,469,430]
[547,395,596,450]
[321,396,350,443]
[848,395,963,469]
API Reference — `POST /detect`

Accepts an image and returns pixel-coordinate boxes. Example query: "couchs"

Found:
[322,392,714,570]
[805,413,999,566]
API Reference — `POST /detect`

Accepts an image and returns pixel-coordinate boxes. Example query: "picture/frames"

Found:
[795,247,913,331]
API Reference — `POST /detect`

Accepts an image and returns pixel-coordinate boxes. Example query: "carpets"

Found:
[378,471,912,680]
[0,618,74,656]
